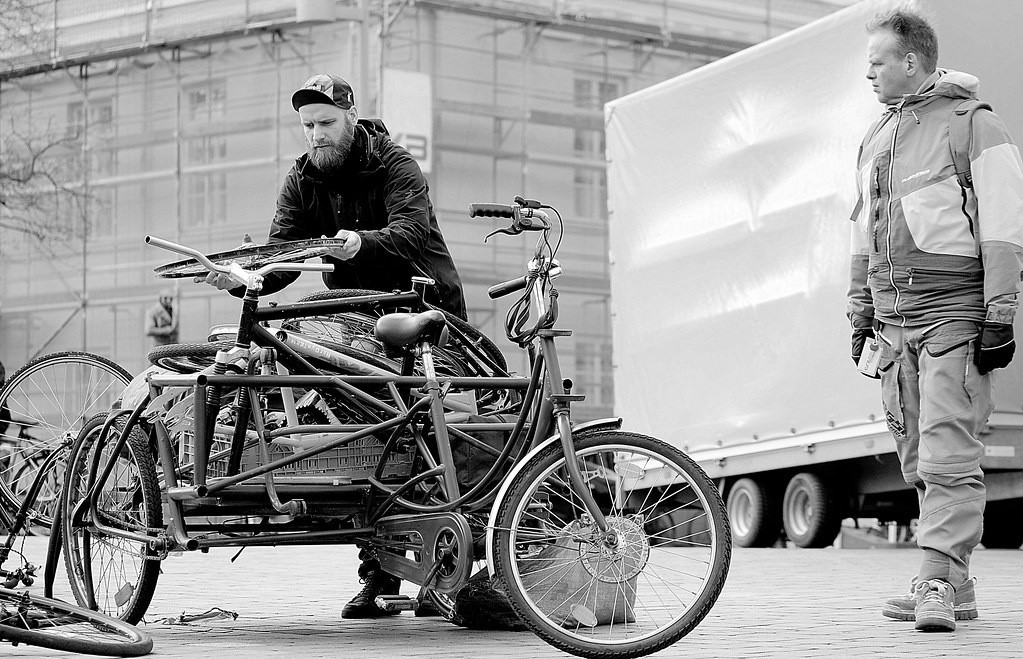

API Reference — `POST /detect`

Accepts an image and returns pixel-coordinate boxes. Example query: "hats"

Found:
[292,74,352,111]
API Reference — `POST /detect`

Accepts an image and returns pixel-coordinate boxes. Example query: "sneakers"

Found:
[342,572,401,622]
[415,593,439,618]
[914,577,954,631]
[884,577,978,620]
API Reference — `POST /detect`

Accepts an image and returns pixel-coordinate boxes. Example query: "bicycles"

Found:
[0,349,155,657]
[57,195,733,659]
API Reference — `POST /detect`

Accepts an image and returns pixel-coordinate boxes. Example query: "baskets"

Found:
[178,430,416,485]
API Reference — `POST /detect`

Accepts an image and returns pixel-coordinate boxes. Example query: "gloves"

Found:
[852,327,881,381]
[974,320,1015,375]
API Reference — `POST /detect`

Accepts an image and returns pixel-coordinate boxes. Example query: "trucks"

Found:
[602,0,1023,551]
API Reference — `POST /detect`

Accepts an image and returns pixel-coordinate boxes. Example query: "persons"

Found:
[846,11,1023,630]
[205,74,468,618]
